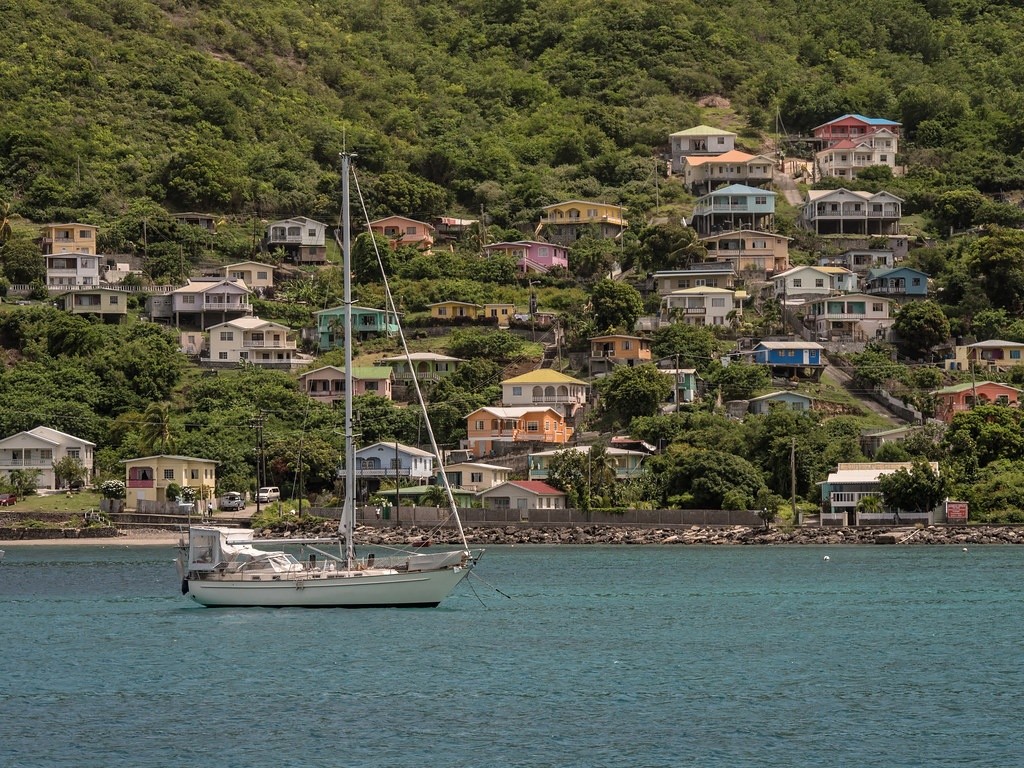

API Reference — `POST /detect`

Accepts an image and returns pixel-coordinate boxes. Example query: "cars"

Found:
[0,494,17,507]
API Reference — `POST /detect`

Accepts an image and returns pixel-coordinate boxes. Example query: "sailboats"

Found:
[183,131,487,608]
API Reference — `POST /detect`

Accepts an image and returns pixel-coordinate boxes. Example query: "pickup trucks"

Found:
[220,495,246,511]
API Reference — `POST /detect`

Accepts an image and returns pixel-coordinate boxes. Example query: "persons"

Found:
[375,506,381,519]
[207,502,212,517]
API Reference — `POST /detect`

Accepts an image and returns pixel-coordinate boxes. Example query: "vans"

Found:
[255,487,280,503]
